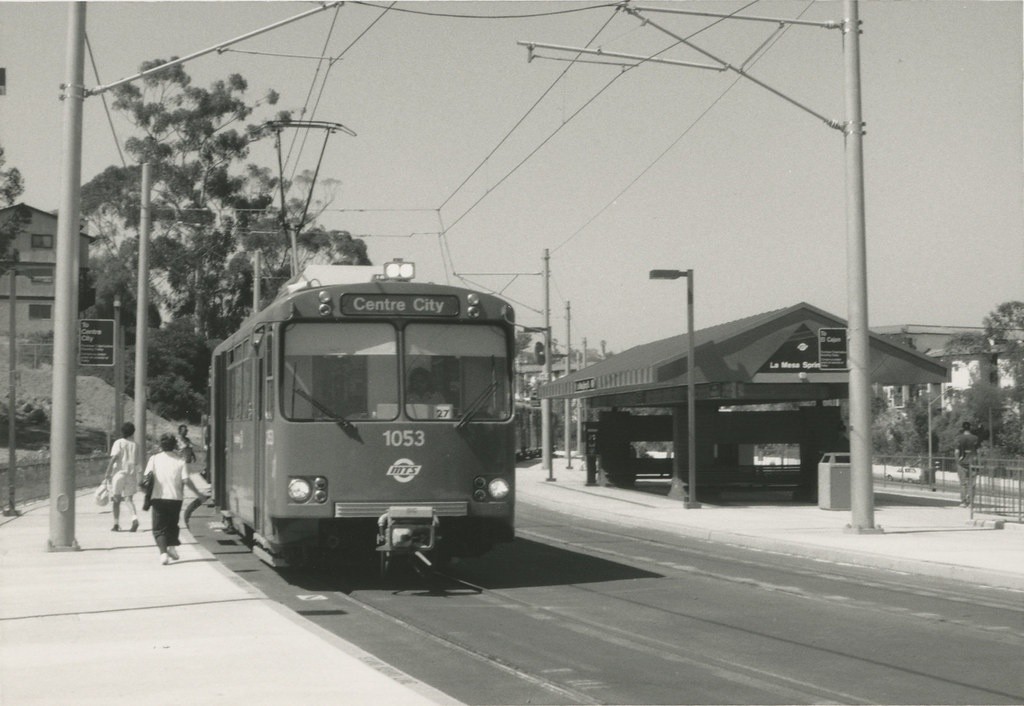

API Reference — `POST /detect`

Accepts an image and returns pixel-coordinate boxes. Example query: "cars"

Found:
[885,466,924,484]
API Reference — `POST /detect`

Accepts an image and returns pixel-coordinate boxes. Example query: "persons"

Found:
[144,433,206,565]
[954,422,979,507]
[405,367,446,404]
[176,425,197,463]
[105,422,142,532]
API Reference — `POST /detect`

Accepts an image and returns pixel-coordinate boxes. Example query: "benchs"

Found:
[681,463,801,492]
[601,458,674,479]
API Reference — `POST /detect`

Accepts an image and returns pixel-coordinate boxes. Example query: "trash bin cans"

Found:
[817,452,850,511]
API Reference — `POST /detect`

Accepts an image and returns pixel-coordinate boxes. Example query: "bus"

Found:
[515,401,556,464]
[200,118,548,571]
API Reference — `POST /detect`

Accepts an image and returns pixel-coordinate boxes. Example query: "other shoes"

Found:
[112,525,118,531]
[162,554,168,565]
[131,520,138,531]
[167,546,179,560]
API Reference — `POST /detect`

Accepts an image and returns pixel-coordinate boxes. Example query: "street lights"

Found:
[552,352,574,470]
[520,324,556,482]
[649,267,702,510]
[988,404,1012,497]
[926,384,964,487]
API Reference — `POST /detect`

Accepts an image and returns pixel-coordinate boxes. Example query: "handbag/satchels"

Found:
[140,456,156,510]
[96,479,110,506]
